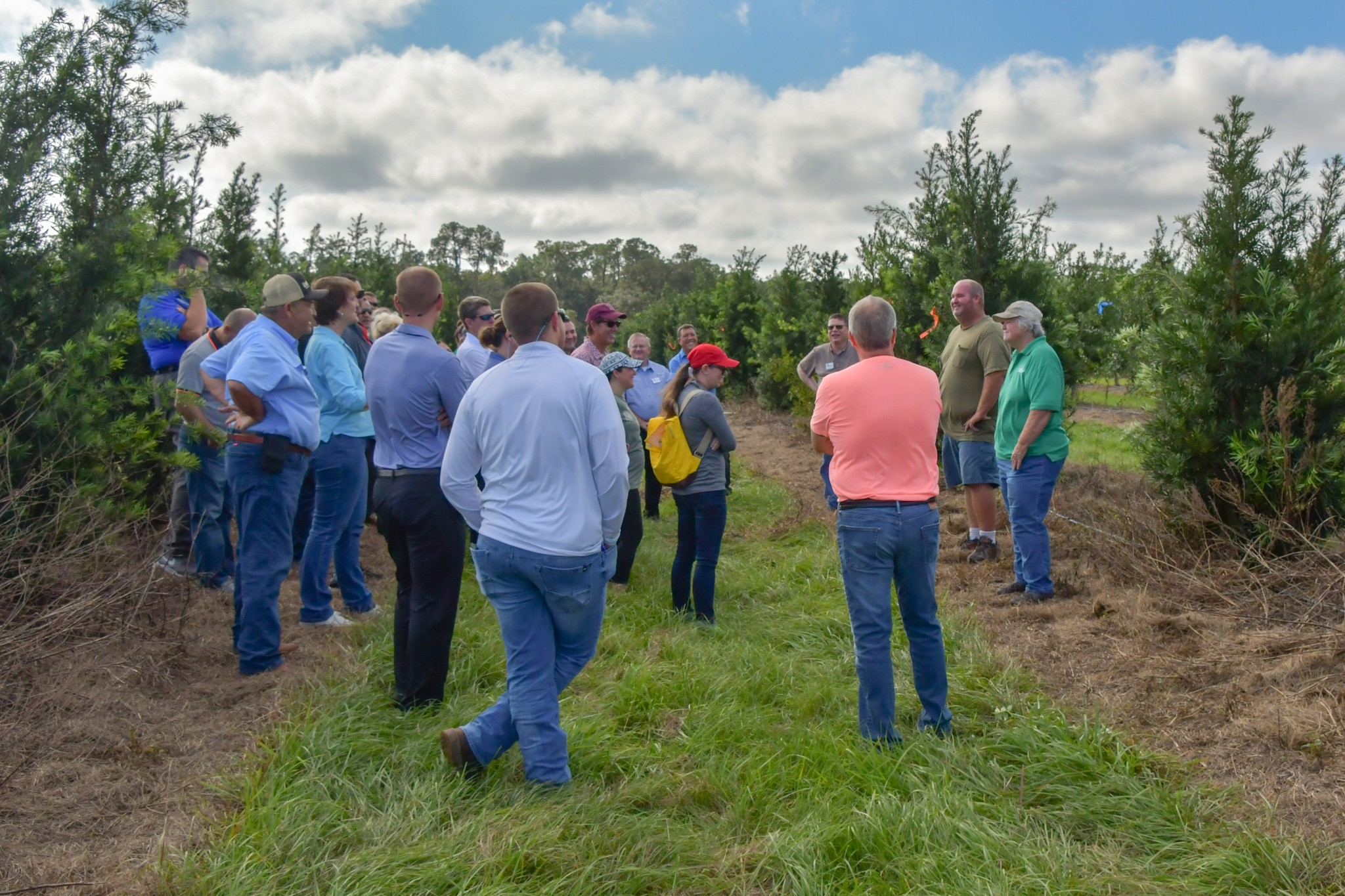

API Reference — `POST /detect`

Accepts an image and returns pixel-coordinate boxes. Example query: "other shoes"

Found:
[328,561,387,591]
[258,661,311,676]
[723,487,733,496]
[298,610,356,628]
[996,582,1025,594]
[232,642,300,657]
[440,727,484,786]
[353,605,392,621]
[211,582,235,593]
[1009,591,1053,605]
[643,510,660,522]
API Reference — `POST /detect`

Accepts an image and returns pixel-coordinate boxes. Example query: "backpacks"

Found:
[644,389,713,489]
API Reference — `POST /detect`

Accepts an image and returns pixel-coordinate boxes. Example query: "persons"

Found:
[796,314,860,510]
[137,248,738,626]
[811,296,953,750]
[362,266,466,710]
[992,301,1071,606]
[441,282,629,792]
[939,278,1012,562]
[200,271,321,677]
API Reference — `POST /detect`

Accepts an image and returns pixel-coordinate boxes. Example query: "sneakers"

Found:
[967,535,998,562]
[959,536,977,552]
[155,549,198,577]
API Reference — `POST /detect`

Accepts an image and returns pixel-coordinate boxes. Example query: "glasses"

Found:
[476,314,494,320]
[599,319,622,328]
[544,308,567,324]
[827,325,847,329]
[369,302,378,307]
[356,308,373,315]
[695,365,726,372]
[353,288,366,299]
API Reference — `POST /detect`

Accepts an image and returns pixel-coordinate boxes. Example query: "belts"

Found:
[378,467,441,476]
[153,363,179,375]
[838,497,936,511]
[228,432,311,458]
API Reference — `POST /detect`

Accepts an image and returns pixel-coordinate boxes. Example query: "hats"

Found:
[585,303,627,325]
[262,272,329,308]
[687,343,740,367]
[992,300,1043,323]
[599,351,645,375]
